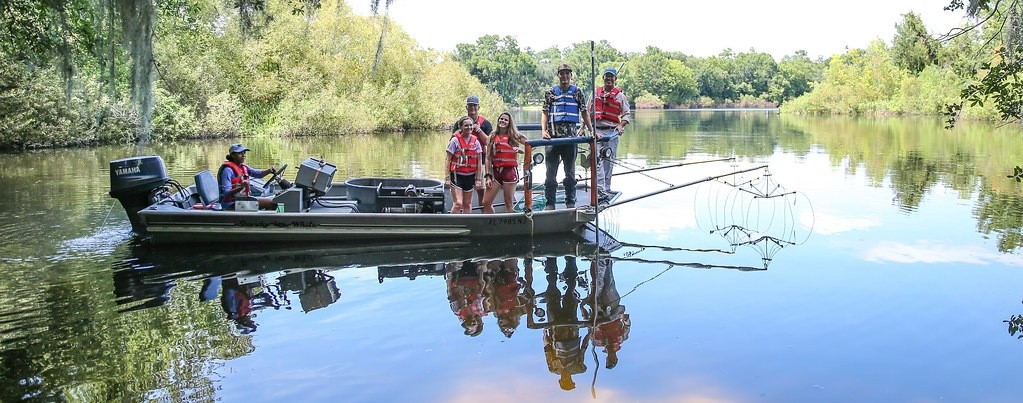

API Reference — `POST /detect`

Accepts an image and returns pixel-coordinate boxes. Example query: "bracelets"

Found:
[477,129,482,133]
[484,174,492,178]
[445,176,451,180]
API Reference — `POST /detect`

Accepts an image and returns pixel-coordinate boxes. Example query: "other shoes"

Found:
[545,204,555,210]
[566,202,576,208]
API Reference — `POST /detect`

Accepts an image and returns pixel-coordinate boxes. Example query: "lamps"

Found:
[530,153,544,169]
[599,146,612,160]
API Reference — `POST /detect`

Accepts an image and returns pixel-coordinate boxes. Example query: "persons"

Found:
[221,275,278,334]
[444,116,483,213]
[489,259,529,335]
[482,112,528,214]
[445,259,486,337]
[541,63,602,210]
[543,256,590,390]
[453,96,492,211]
[578,67,631,197]
[582,257,632,368]
[217,144,277,209]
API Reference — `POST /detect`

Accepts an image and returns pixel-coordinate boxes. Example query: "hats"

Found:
[229,144,250,154]
[558,64,572,74]
[467,96,479,105]
[604,67,616,76]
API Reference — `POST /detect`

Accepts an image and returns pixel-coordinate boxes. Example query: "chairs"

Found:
[195,170,224,206]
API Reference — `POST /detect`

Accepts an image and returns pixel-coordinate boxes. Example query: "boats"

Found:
[129,223,622,273]
[108,155,624,246]
